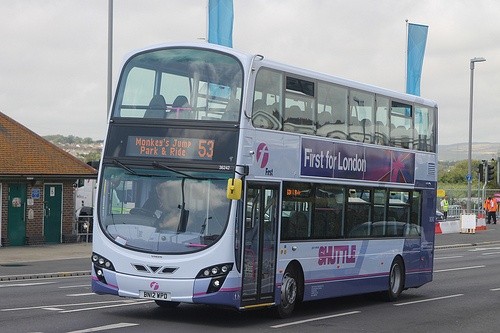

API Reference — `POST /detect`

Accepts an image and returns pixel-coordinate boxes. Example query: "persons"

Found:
[486,194,498,224]
[441,195,449,219]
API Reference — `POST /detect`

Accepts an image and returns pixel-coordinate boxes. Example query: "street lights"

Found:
[467,56,486,217]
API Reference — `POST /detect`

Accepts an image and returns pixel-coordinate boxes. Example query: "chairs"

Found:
[143,95,418,149]
[286,210,420,239]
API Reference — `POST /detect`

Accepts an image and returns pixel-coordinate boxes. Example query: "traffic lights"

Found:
[478,160,495,184]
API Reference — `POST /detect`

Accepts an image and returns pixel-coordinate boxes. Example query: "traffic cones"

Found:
[476,213,487,230]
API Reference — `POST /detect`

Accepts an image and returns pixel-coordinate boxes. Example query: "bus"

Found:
[91,41,439,318]
